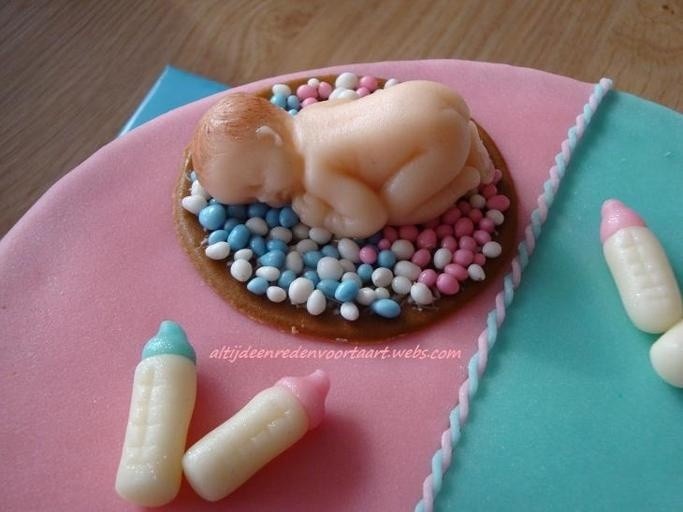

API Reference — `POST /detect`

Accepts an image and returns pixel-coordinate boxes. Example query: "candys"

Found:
[115,71,683,506]
